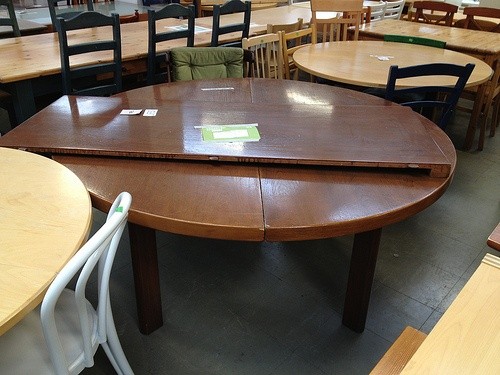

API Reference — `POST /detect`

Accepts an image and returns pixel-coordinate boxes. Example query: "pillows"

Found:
[171,46,245,81]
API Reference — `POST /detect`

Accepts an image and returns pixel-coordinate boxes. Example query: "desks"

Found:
[0,0,243,125]
[0,146,93,336]
[292,0,384,8]
[348,18,500,150]
[179,0,287,11]
[409,5,500,23]
[293,41,494,122]
[184,5,345,37]
[370,253,500,375]
[0,77,457,335]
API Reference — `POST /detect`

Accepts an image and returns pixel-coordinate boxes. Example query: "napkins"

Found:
[242,0,500,151]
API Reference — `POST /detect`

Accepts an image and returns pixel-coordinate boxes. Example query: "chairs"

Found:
[211,0,252,48]
[166,47,255,83]
[364,63,476,130]
[48,0,94,33]
[148,3,195,85]
[55,11,122,96]
[0,0,21,39]
[0,192,135,375]
